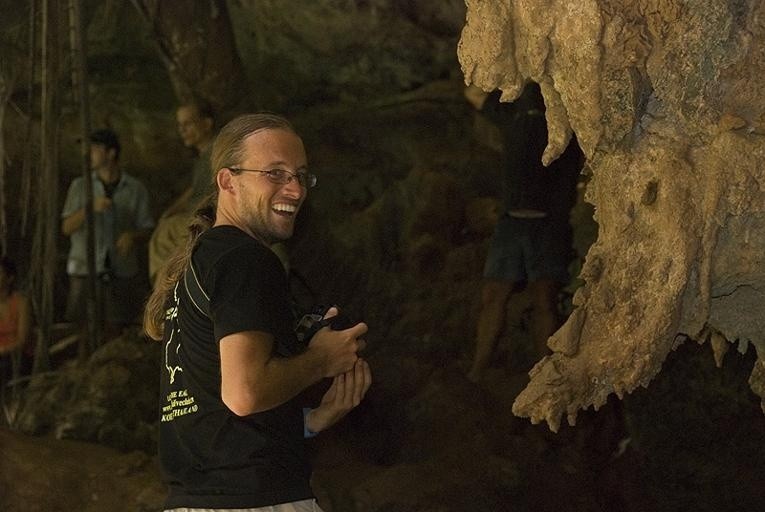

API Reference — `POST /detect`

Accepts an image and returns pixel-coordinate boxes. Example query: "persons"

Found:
[460,82,584,382]
[0,257,38,404]
[147,98,221,289]
[141,111,372,512]
[61,125,155,344]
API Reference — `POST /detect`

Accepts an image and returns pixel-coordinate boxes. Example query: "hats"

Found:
[78,131,120,150]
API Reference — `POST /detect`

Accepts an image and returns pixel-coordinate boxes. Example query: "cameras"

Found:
[294,301,356,346]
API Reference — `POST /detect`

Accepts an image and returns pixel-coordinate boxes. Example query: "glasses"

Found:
[230,168,318,188]
